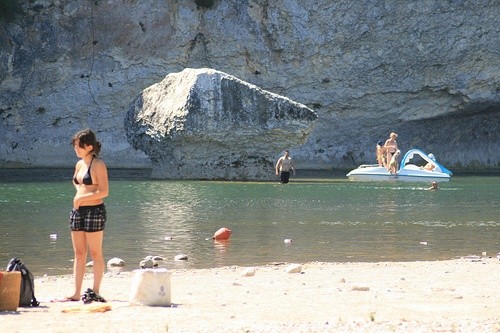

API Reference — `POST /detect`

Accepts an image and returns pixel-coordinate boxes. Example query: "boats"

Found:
[345,149,453,183]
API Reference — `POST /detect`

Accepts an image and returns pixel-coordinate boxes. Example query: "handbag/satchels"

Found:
[7,257,40,306]
[129,267,172,306]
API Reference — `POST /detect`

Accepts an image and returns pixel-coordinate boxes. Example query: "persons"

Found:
[275,150,296,183]
[422,152,436,172]
[63,127,109,303]
[376,132,401,173]
